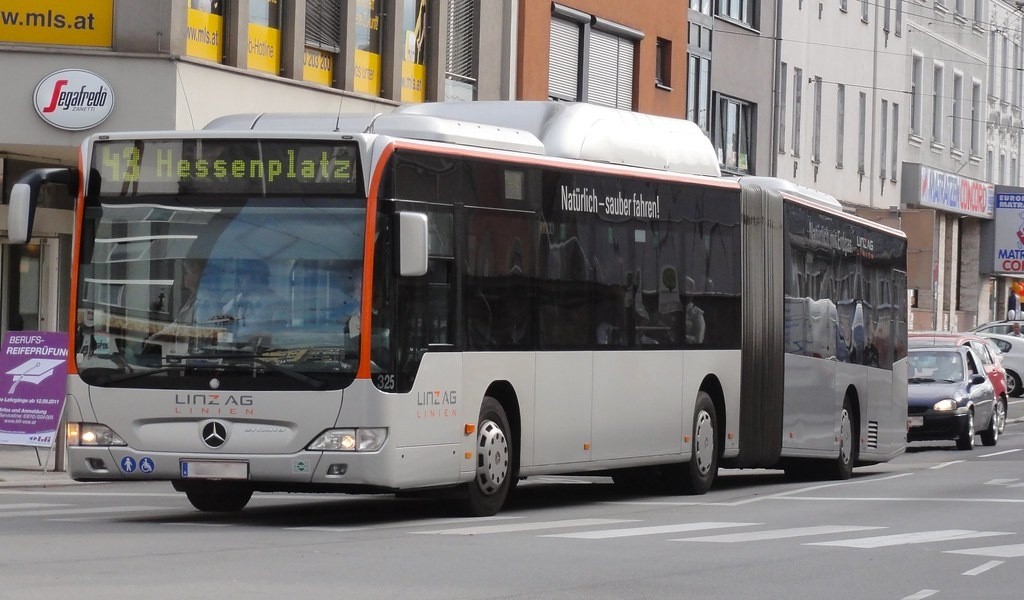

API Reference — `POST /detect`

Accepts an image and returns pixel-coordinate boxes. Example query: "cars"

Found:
[961,332,1024,400]
[908,347,998,452]
[906,335,1008,435]
[968,318,1024,338]
[948,332,1004,370]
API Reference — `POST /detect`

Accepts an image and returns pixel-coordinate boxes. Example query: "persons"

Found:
[1006,322,1024,338]
[213,260,288,341]
[658,277,707,347]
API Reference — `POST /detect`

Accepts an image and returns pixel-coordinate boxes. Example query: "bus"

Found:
[6,100,907,519]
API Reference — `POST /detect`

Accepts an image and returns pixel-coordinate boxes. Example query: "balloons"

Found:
[1008,310,1015,320]
[1012,282,1024,302]
[1015,311,1024,320]
